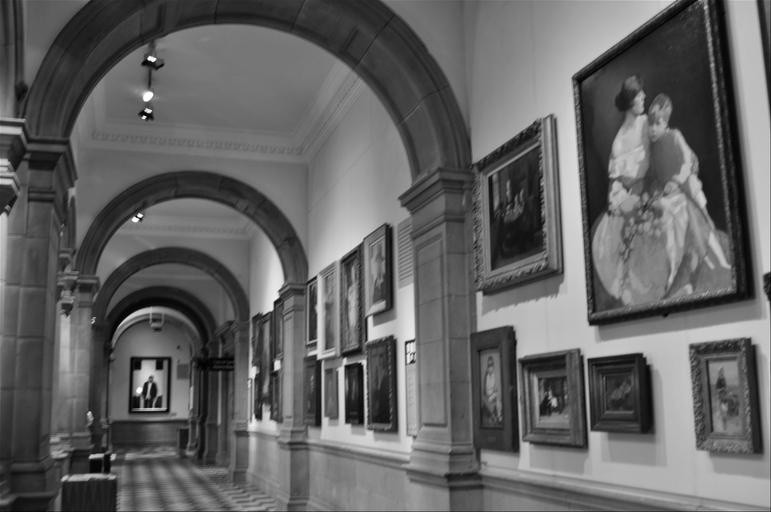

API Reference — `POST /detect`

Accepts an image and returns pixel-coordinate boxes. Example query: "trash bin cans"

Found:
[177,429,189,449]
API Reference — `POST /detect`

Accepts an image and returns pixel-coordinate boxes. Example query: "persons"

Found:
[481,356,502,426]
[714,367,740,427]
[278,241,388,431]
[141,374,157,408]
[498,180,534,255]
[539,381,560,417]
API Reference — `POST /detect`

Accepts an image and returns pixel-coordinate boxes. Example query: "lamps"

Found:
[136,37,165,119]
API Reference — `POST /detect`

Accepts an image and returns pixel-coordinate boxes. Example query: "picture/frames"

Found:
[688,338,760,454]
[469,114,564,295]
[519,348,585,447]
[588,352,646,434]
[470,325,520,453]
[128,356,171,413]
[250,223,398,432]
[571,0,755,326]
